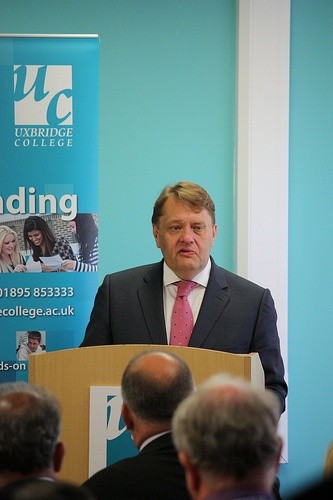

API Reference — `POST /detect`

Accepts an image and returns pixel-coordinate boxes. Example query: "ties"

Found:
[167,281,198,347]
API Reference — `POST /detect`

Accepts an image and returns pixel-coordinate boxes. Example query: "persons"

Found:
[0,381,90,500]
[80,351,281,500]
[79,181,288,414]
[17,331,46,361]
[23,216,77,272]
[61,213,98,272]
[0,477,92,500]
[0,225,27,273]
[172,374,283,500]
